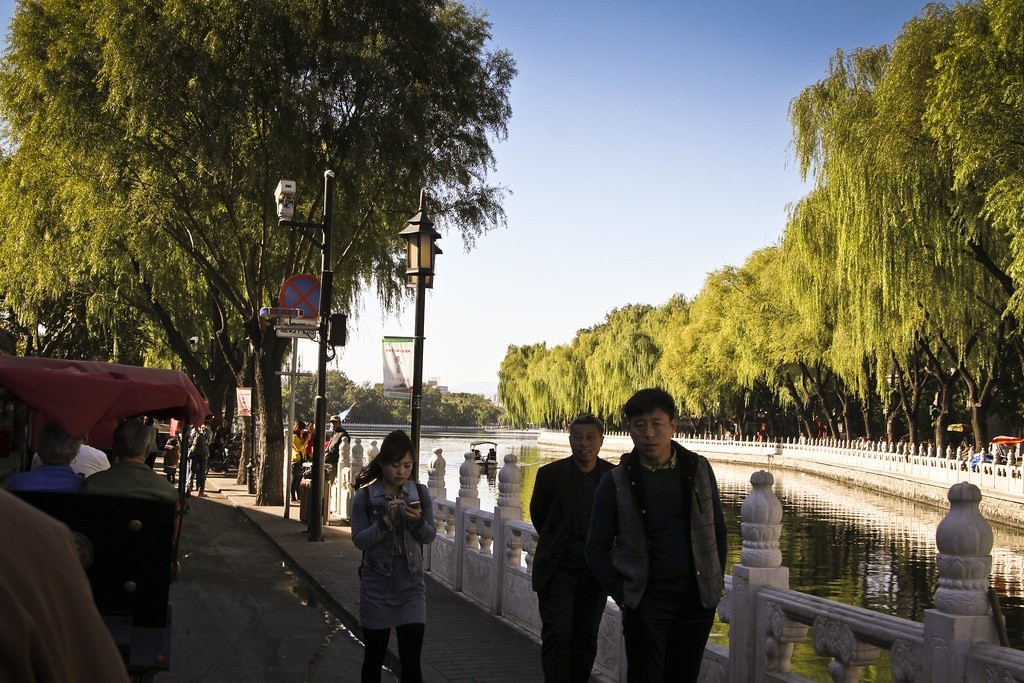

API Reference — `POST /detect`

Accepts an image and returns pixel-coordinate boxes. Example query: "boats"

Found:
[469,440,498,469]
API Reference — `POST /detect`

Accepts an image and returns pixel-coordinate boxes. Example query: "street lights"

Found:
[273,169,347,543]
[397,185,443,487]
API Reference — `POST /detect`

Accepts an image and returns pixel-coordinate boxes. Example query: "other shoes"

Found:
[196,489,198,491]
[198,492,207,497]
[172,481,175,484]
[291,499,299,504]
[187,487,191,495]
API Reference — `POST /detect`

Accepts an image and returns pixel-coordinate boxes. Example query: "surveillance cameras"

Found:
[274,179,297,200]
[189,336,198,345]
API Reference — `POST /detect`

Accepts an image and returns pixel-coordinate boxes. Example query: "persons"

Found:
[728,429,1017,476]
[529,415,619,683]
[472,448,496,460]
[349,430,438,683]
[585,387,727,683]
[0,401,241,683]
[291,415,350,520]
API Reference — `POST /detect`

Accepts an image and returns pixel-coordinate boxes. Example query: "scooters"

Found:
[208,433,243,472]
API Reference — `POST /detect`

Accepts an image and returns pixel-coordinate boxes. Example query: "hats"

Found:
[329,415,341,422]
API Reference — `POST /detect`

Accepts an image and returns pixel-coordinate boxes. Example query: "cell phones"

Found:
[407,501,421,509]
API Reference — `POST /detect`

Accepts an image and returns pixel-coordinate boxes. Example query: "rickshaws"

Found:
[0,354,214,683]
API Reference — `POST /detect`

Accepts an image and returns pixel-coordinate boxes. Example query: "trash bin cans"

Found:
[299,462,333,525]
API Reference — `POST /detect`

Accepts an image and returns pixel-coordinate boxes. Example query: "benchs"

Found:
[5,488,180,683]
